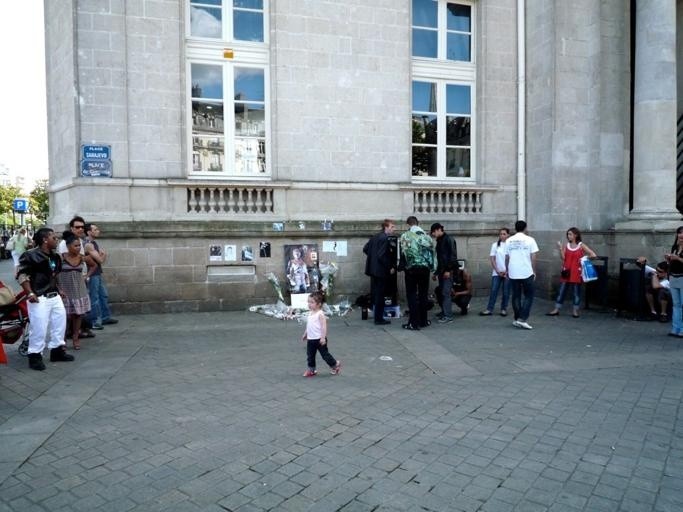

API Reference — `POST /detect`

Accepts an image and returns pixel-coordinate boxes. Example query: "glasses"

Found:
[656,268,663,273]
[74,226,85,229]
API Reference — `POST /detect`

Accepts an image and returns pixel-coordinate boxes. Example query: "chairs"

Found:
[582,256,616,313]
[613,258,660,322]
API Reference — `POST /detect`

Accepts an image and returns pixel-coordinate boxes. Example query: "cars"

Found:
[0,230,33,259]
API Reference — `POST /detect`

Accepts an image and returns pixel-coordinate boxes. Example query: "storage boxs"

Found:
[373,304,400,319]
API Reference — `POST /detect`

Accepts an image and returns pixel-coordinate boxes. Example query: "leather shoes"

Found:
[669,333,676,336]
[676,335,683,338]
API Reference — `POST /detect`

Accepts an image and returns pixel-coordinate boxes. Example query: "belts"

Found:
[41,292,58,298]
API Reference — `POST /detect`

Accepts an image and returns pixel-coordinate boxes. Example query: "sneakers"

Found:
[331,361,341,375]
[660,314,669,323]
[375,307,579,330]
[645,313,657,320]
[303,369,317,377]
[29,318,118,370]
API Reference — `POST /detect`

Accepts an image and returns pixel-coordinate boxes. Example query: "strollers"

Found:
[0,290,30,357]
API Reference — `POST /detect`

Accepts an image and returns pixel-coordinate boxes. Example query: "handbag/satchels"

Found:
[5,241,15,250]
[561,268,570,277]
[580,256,599,282]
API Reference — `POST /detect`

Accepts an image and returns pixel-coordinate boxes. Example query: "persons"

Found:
[0,216,119,371]
[430,223,457,323]
[478,229,510,317]
[663,225,682,338]
[635,256,673,321]
[434,266,473,317]
[395,216,436,330]
[361,219,395,324]
[504,221,539,330]
[302,292,341,378]
[543,227,595,318]
[285,248,309,294]
